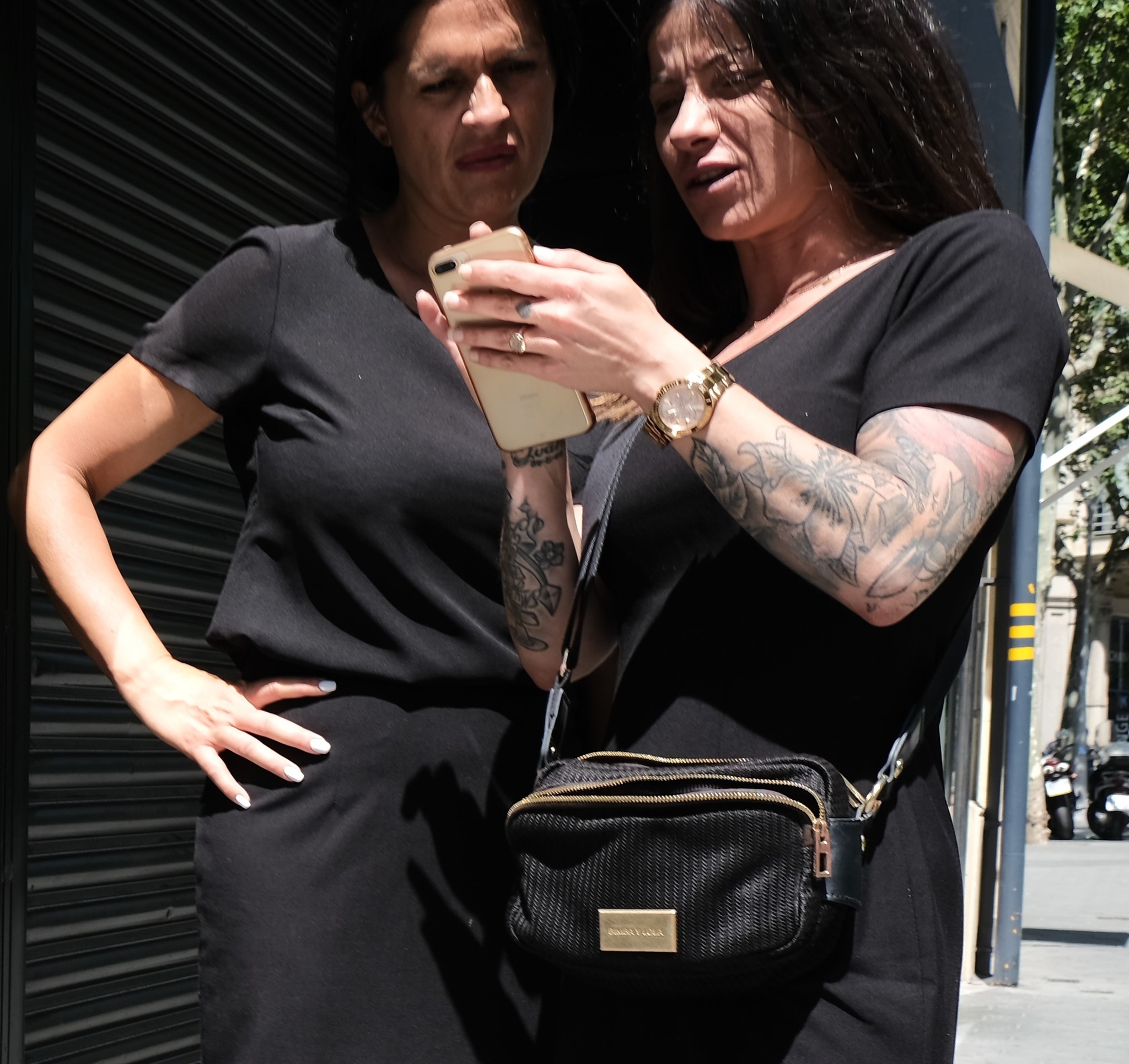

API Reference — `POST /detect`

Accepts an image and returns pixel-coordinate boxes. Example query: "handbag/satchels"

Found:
[504,754,868,969]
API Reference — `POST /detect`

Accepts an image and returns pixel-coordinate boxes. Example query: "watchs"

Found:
[643,361,736,450]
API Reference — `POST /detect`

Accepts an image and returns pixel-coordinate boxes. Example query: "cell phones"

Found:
[427,225,600,453]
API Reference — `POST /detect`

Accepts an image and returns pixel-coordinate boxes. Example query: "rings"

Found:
[509,324,528,355]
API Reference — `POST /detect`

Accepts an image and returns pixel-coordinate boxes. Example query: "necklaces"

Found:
[744,245,867,334]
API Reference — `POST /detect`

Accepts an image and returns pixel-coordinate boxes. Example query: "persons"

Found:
[11,0,620,1064]
[415,0,1073,1064]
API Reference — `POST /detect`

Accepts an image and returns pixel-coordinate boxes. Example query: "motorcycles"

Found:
[1039,728,1129,841]
